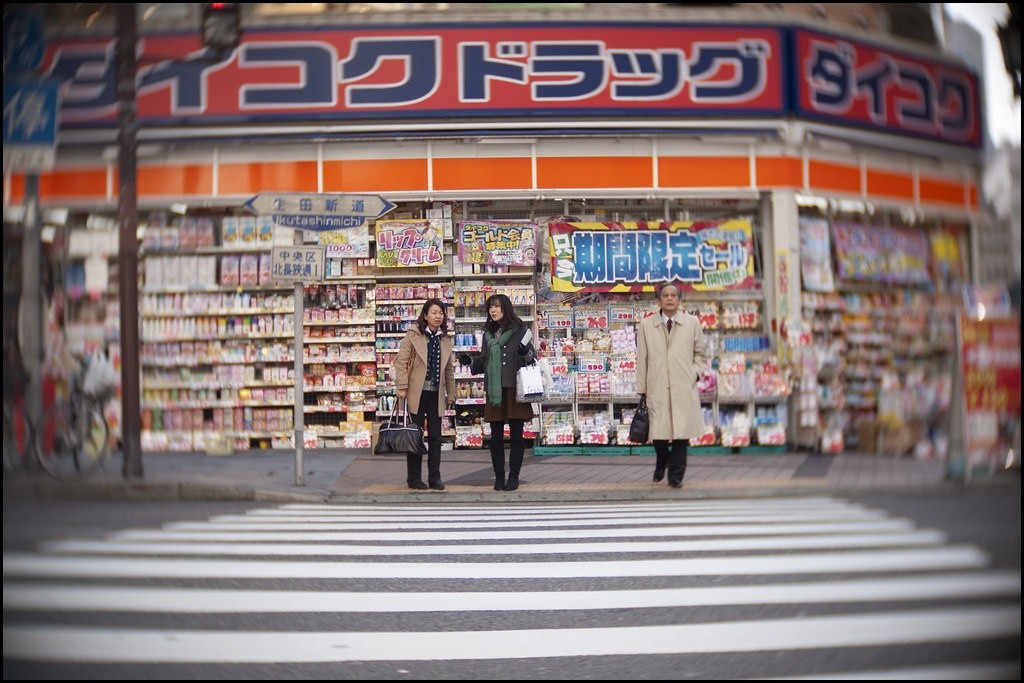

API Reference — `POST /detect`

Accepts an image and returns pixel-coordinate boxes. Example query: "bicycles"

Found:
[0,354,41,484]
[36,325,114,478]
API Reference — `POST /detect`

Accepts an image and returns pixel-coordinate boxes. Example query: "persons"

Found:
[460,294,536,491]
[635,282,707,489]
[394,298,456,490]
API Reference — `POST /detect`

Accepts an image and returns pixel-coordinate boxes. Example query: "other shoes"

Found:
[408,481,427,490]
[505,479,521,490]
[653,458,669,482]
[428,478,445,490]
[494,478,507,490]
[671,474,684,488]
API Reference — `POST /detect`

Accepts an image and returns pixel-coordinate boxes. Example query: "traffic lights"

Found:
[197,0,245,48]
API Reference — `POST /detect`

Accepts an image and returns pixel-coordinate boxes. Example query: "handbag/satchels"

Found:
[516,358,555,403]
[374,396,428,455]
[629,394,650,444]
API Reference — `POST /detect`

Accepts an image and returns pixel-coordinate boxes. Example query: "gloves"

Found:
[519,343,529,355]
[459,354,472,367]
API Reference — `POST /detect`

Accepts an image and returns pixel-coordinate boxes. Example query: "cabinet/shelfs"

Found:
[64,199,977,454]
